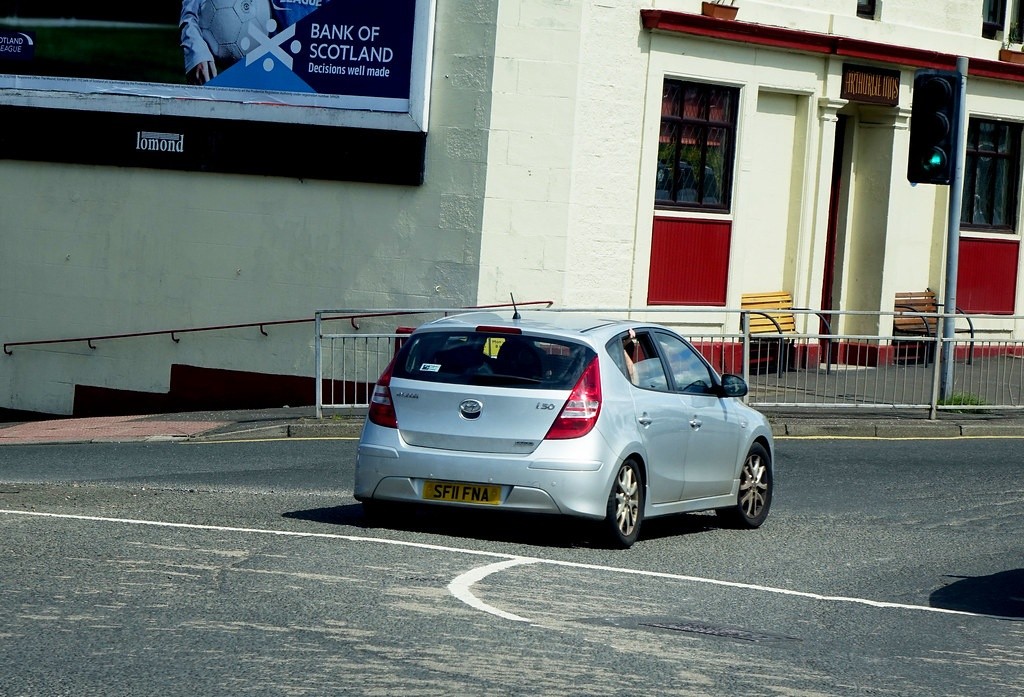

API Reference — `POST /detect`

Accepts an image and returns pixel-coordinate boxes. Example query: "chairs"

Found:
[496,338,543,380]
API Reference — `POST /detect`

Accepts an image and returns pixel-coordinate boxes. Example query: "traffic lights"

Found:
[906,68,962,186]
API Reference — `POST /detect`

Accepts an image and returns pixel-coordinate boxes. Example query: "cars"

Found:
[354,309,776,549]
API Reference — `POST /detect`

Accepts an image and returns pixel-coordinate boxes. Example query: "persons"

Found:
[624,328,640,387]
[177,0,218,83]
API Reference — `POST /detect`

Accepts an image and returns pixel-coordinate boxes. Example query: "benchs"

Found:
[895,288,975,367]
[742,292,833,377]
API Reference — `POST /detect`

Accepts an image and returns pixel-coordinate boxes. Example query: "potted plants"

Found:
[703,0,740,20]
[999,21,1024,64]
